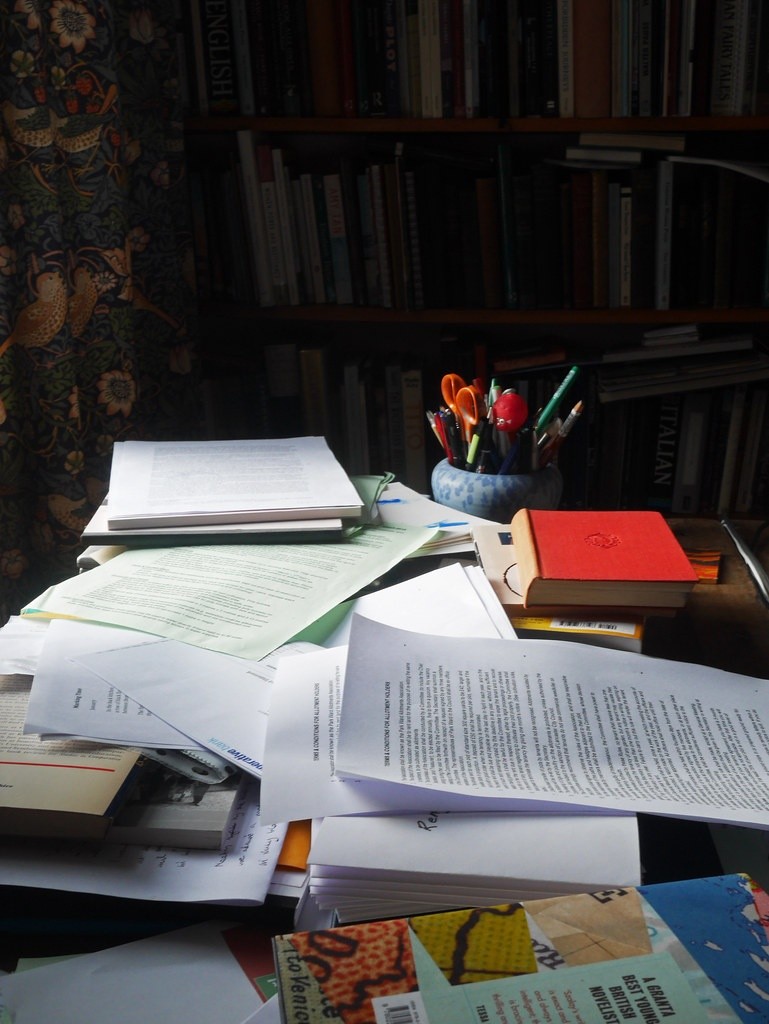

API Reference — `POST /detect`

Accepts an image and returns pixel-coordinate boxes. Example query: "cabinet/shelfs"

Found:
[186,117,769,520]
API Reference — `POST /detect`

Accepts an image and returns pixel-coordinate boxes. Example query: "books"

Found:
[172,1,763,119]
[80,437,364,543]
[0,673,243,848]
[255,873,769,1024]
[188,131,768,308]
[200,335,769,514]
[470,509,722,653]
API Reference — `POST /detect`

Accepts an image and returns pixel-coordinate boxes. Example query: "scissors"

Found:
[440,372,483,458]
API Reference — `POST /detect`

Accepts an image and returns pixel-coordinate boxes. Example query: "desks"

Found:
[0,516,769,973]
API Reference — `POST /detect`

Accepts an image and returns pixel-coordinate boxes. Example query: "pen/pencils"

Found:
[429,364,586,474]
